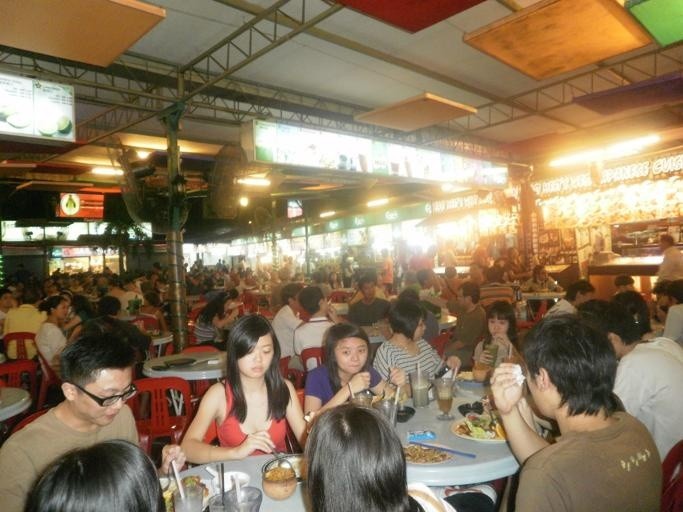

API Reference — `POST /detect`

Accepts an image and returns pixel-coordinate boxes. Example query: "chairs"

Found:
[125,377,194,457]
[329,291,352,303]
[10,407,53,435]
[660,440,683,512]
[31,339,65,412]
[296,386,308,417]
[2,331,40,363]
[130,317,158,362]
[163,343,175,358]
[301,348,328,374]
[203,419,219,446]
[430,331,452,357]
[514,298,548,331]
[0,359,38,395]
[181,344,221,400]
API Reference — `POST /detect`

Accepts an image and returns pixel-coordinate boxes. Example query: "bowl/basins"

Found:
[457,402,485,417]
[211,471,250,494]
[396,405,416,424]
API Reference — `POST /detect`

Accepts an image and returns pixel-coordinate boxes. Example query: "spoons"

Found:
[163,460,172,493]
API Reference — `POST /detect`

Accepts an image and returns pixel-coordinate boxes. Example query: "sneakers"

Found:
[428,482,499,507]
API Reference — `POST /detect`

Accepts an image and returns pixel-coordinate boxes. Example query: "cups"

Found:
[378,320,384,328]
[434,378,456,420]
[484,344,498,368]
[441,304,448,321]
[173,485,204,512]
[374,400,397,431]
[207,493,225,512]
[410,371,431,408]
[224,487,263,512]
[349,392,372,407]
[372,323,381,336]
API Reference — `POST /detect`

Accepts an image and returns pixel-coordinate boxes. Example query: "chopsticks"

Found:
[409,441,477,458]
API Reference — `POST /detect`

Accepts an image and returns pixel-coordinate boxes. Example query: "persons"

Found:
[273,283,305,372]
[663,279,683,346]
[443,281,487,367]
[303,322,405,424]
[543,280,595,319]
[24,439,167,511]
[465,234,522,285]
[299,404,457,512]
[373,302,461,398]
[472,301,517,376]
[138,291,168,333]
[0,334,140,512]
[0,261,170,303]
[193,292,239,351]
[3,288,47,359]
[524,264,563,312]
[0,288,12,340]
[479,267,513,309]
[652,280,672,326]
[180,315,308,464]
[399,288,435,338]
[579,299,682,483]
[613,275,635,296]
[610,291,683,362]
[349,279,390,325]
[648,235,683,294]
[79,296,152,420]
[183,239,464,289]
[576,299,609,326]
[490,314,663,512]
[34,295,82,379]
[294,286,344,371]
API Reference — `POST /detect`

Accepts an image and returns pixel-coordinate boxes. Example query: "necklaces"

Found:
[391,338,411,350]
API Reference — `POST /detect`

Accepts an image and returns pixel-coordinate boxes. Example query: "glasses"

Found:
[439,366,450,376]
[72,383,138,408]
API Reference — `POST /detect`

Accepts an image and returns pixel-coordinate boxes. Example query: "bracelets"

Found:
[346,381,355,399]
[210,445,218,463]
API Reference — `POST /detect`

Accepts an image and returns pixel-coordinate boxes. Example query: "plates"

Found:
[261,452,312,486]
[400,442,454,466]
[450,415,509,444]
